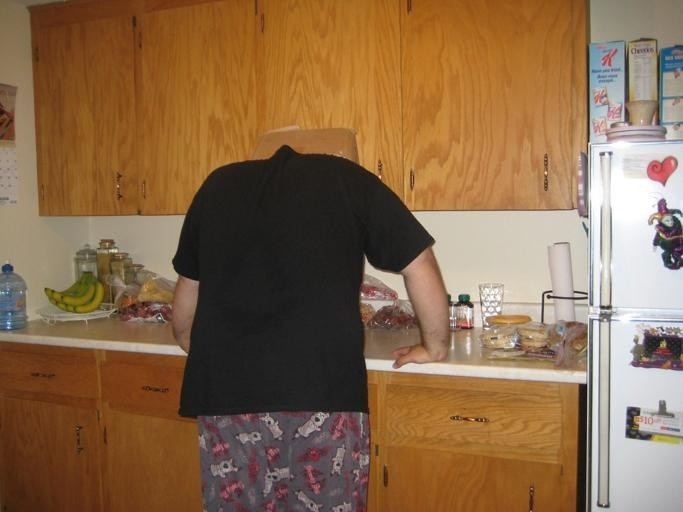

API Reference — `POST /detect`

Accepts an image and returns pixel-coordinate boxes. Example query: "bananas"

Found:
[44,272,105,313]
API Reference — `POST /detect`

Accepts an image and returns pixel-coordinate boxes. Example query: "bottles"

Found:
[447,293,474,329]
[73,239,144,304]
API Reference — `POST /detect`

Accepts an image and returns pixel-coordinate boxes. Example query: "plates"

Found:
[36,306,106,317]
[584,140,682,511]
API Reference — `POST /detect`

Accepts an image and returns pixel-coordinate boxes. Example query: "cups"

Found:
[478,282,504,331]
[625,99,659,125]
[514,328,551,352]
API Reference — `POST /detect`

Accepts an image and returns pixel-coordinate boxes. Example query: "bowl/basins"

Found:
[605,126,667,141]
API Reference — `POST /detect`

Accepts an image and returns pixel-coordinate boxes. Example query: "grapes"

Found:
[642,329,664,359]
[666,337,683,359]
[626,407,649,440]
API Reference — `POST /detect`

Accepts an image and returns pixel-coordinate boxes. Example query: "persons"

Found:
[171,145,452,511]
